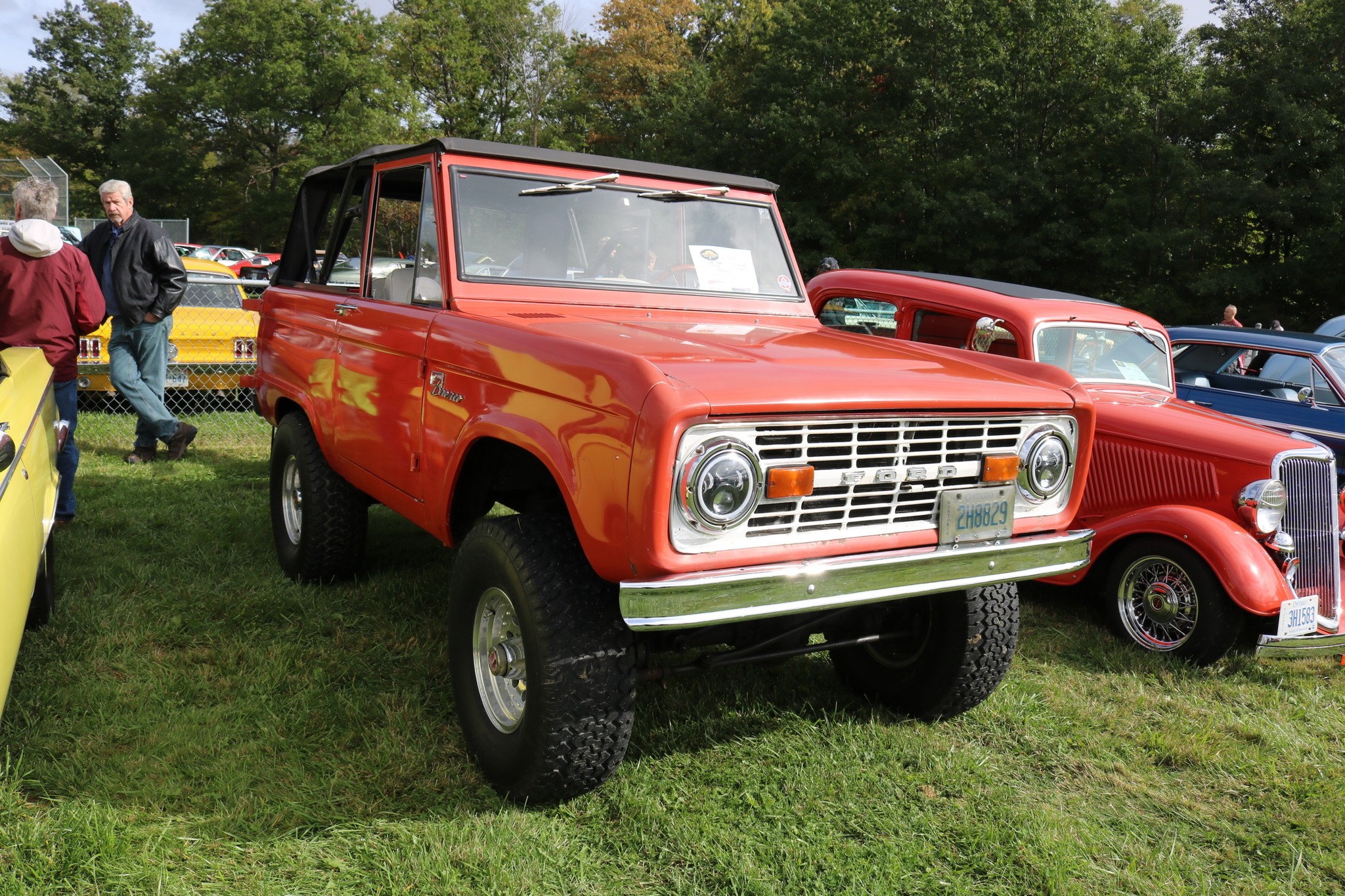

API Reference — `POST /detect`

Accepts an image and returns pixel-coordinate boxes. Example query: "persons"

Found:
[632,249,664,287]
[1220,304,1245,376]
[817,257,840,275]
[590,236,620,278]
[1270,320,1281,331]
[0,176,106,536]
[73,179,198,466]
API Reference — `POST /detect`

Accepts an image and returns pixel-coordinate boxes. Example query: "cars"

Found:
[803,266,1345,670]
[171,242,653,295]
[77,256,261,400]
[1038,323,1345,502]
[0,345,70,725]
[1258,313,1345,389]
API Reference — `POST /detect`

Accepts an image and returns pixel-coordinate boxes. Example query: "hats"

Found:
[1272,320,1280,328]
[819,257,839,271]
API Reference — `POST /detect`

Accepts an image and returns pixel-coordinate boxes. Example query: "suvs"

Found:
[238,136,1098,807]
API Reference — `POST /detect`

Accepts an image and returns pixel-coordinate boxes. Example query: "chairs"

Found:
[1175,370,1210,387]
[1260,388,1299,401]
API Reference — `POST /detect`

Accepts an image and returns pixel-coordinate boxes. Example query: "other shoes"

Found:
[127,446,157,464]
[167,420,197,461]
